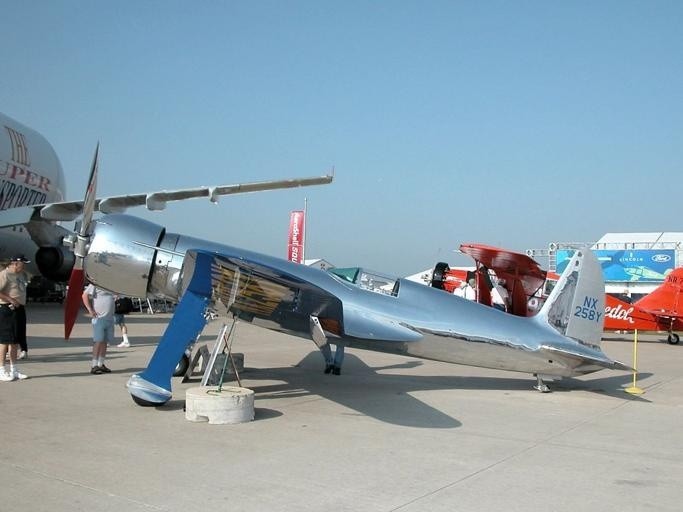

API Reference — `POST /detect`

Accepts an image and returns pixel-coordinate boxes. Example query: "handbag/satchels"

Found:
[115,297,133,314]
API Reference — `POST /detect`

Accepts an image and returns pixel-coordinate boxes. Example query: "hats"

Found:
[10,253,31,264]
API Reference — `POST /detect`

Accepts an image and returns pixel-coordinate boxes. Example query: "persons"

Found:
[113,294,131,348]
[0,251,33,383]
[0,261,28,362]
[490,278,509,313]
[82,283,115,374]
[617,291,631,334]
[466,278,476,300]
[454,281,466,298]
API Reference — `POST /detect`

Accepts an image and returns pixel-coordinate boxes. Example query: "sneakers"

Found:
[117,342,130,348]
[0,369,28,381]
[17,350,27,360]
[90,364,111,374]
